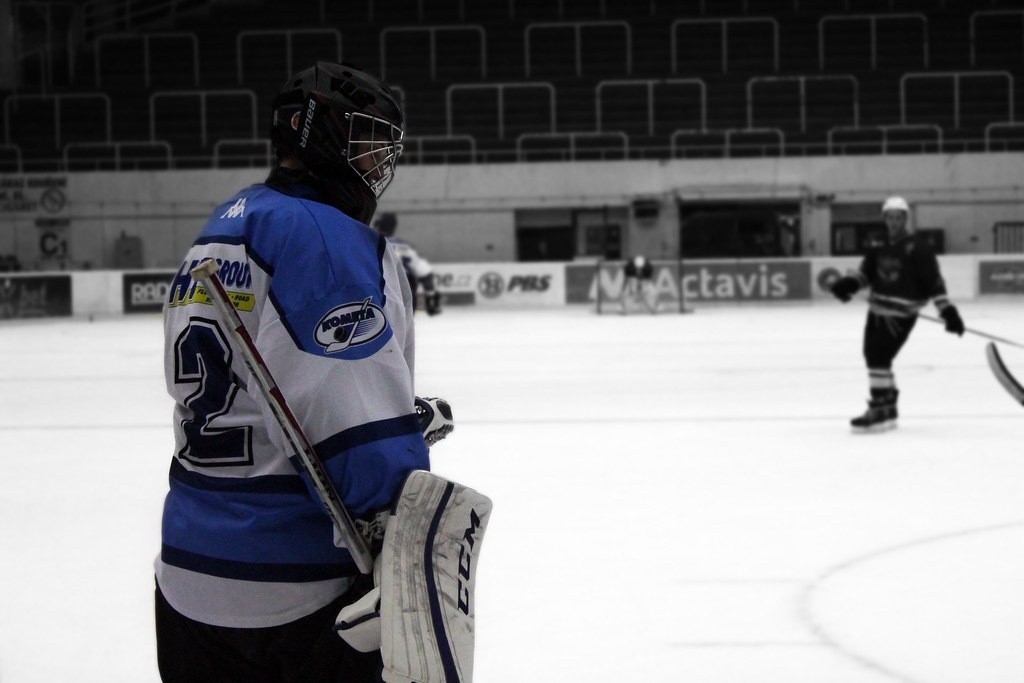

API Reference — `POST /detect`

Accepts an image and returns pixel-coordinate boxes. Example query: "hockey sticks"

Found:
[851,291,1023,351]
[985,340,1024,408]
[188,254,376,579]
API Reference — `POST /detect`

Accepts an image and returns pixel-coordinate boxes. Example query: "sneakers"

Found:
[850,389,898,433]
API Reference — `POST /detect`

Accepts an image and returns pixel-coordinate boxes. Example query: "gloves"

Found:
[423,291,440,316]
[411,395,454,445]
[940,304,964,336]
[831,275,861,302]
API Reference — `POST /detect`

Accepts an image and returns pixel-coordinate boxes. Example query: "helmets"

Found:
[634,256,645,268]
[880,196,910,214]
[267,61,406,223]
[374,212,398,236]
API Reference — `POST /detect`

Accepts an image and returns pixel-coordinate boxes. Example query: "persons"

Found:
[156,60,494,683]
[371,210,443,318]
[831,197,965,434]
[619,256,659,314]
